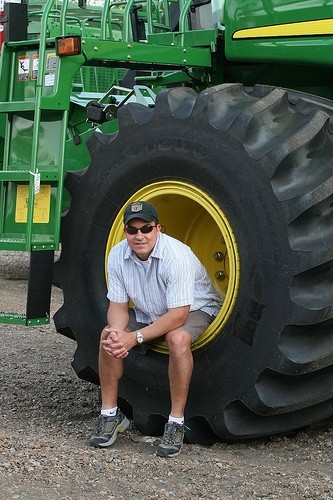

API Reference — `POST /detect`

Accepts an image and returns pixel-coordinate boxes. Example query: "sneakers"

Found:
[90,409,132,447]
[156,416,192,457]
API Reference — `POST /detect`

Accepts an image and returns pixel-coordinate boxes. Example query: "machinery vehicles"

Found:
[1,0,333,447]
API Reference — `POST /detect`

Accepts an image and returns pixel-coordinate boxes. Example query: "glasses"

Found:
[126,224,156,235]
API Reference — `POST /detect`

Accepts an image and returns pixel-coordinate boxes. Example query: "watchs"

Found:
[134,330,144,345]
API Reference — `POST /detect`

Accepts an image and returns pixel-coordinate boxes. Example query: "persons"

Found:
[88,201,225,458]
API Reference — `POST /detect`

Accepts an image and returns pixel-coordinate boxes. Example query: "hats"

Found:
[123,201,159,226]
